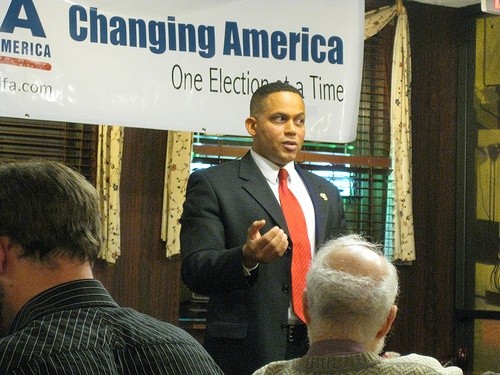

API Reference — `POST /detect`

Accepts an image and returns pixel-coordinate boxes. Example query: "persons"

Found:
[178,82,347,375]
[0,160,225,375]
[251,234,464,375]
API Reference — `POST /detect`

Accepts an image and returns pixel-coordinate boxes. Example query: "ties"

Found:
[278,168,312,326]
